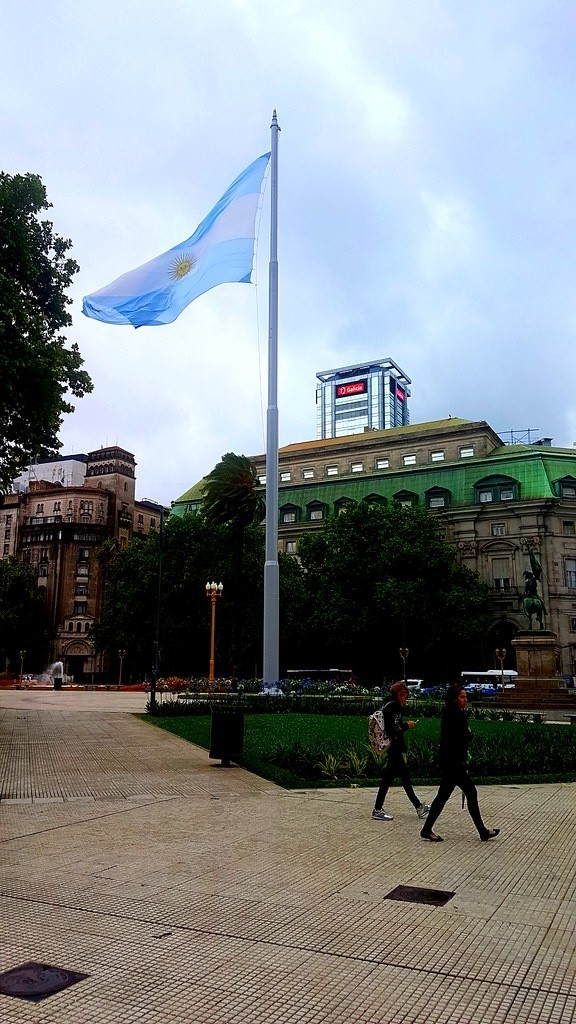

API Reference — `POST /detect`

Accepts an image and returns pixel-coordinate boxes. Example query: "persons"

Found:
[53,658,63,690]
[523,570,549,615]
[372,683,432,821]
[420,686,500,842]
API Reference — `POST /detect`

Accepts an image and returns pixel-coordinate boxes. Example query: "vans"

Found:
[394,679,423,691]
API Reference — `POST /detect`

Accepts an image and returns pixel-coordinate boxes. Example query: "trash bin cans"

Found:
[209,702,245,767]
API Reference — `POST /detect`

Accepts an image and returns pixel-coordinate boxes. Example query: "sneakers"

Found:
[417,803,432,818]
[372,808,394,820]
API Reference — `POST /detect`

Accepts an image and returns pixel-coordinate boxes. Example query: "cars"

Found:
[22,673,49,681]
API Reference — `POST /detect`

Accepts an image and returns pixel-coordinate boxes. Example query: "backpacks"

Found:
[368,700,394,758]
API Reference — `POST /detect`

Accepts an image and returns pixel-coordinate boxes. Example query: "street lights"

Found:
[118,649,126,685]
[496,648,507,694]
[399,647,409,680]
[205,581,223,683]
[19,651,26,682]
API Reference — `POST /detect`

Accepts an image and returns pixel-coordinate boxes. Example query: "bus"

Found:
[285,669,353,689]
[460,670,518,696]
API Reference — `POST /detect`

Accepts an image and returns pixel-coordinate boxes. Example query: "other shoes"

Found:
[488,828,501,839]
[420,831,443,841]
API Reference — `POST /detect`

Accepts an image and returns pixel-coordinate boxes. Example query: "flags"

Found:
[528,551,543,584]
[81,152,270,330]
